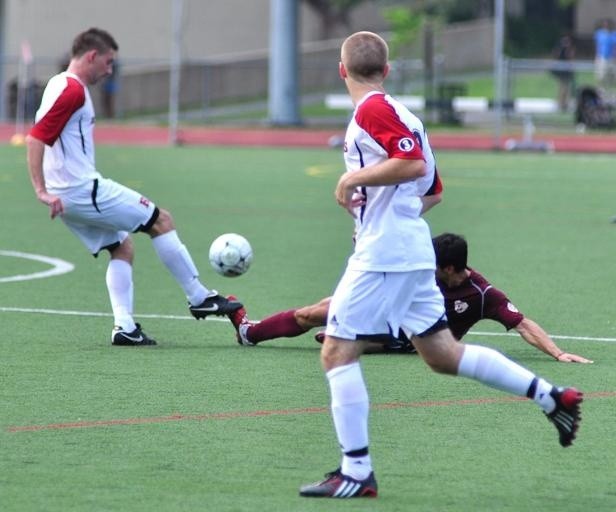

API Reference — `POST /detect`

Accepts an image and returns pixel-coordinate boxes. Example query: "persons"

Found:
[224,233,596,363]
[550,37,574,111]
[592,19,615,86]
[296,31,583,498]
[25,27,242,347]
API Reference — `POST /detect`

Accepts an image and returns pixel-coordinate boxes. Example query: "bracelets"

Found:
[554,351,567,360]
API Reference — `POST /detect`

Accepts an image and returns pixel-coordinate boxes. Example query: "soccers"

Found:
[208,233,252,277]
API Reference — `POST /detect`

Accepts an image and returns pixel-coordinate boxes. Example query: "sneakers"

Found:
[300,467,378,498]
[315,331,325,343]
[111,322,157,346]
[544,386,584,448]
[186,290,256,346]
